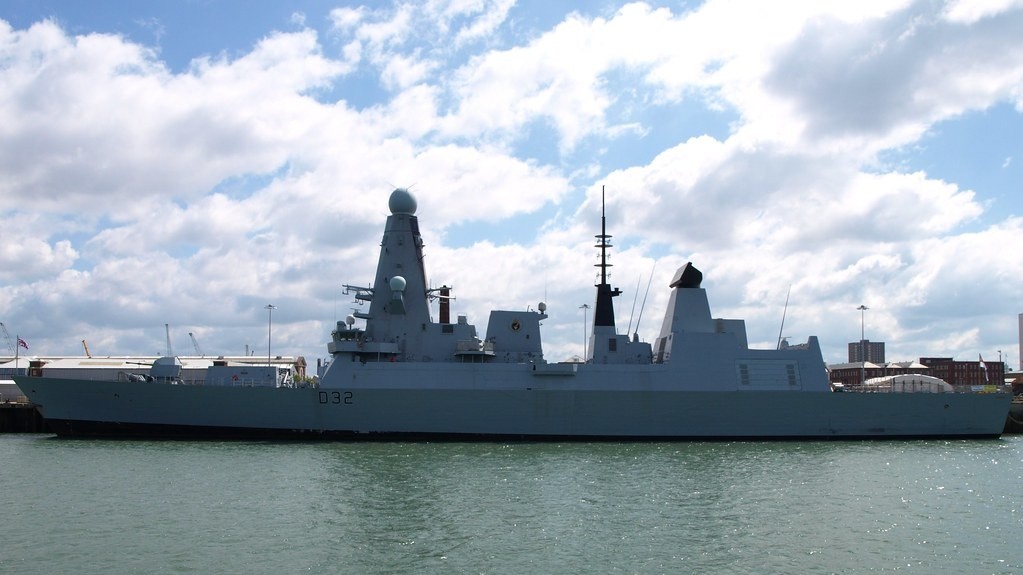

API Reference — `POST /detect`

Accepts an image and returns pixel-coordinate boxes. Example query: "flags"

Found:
[18,337,29,350]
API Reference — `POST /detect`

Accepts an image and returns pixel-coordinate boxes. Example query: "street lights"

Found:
[856,305,868,387]
[264,304,276,368]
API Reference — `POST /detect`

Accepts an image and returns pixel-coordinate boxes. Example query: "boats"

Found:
[10,187,1010,442]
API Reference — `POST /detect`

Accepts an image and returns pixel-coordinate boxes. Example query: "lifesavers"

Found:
[232,375,239,380]
